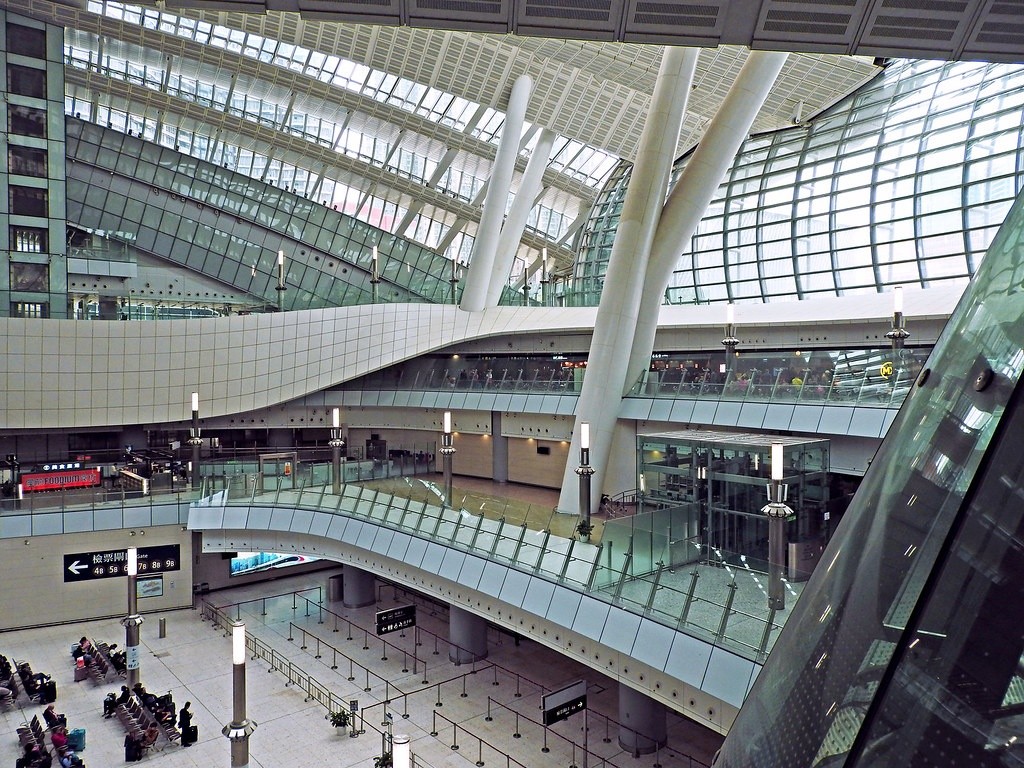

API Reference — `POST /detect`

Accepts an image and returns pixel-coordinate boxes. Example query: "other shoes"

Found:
[105,714,112,719]
[184,744,192,748]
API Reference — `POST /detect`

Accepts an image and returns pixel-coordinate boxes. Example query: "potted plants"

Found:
[329,710,353,737]
[576,519,595,543]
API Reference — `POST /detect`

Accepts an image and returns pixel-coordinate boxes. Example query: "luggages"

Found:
[74,666,87,681]
[69,728,85,752]
[188,726,198,742]
[167,703,175,713]
[125,732,137,761]
[44,681,57,702]
[162,690,172,705]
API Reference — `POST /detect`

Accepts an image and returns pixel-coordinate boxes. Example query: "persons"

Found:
[0,654,51,705]
[111,463,119,487]
[369,443,375,459]
[72,637,126,675]
[105,683,194,762]
[667,452,678,482]
[653,366,912,403]
[417,450,424,463]
[16,705,85,768]
[179,465,190,484]
[392,368,574,391]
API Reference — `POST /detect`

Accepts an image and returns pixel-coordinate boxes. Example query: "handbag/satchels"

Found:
[77,656,84,667]
[142,734,148,741]
[147,697,155,704]
[108,693,115,701]
[68,753,79,762]
[72,647,82,657]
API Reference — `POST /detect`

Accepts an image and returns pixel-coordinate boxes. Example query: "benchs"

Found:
[16,713,78,768]
[114,691,181,760]
[85,636,127,688]
[0,658,48,714]
